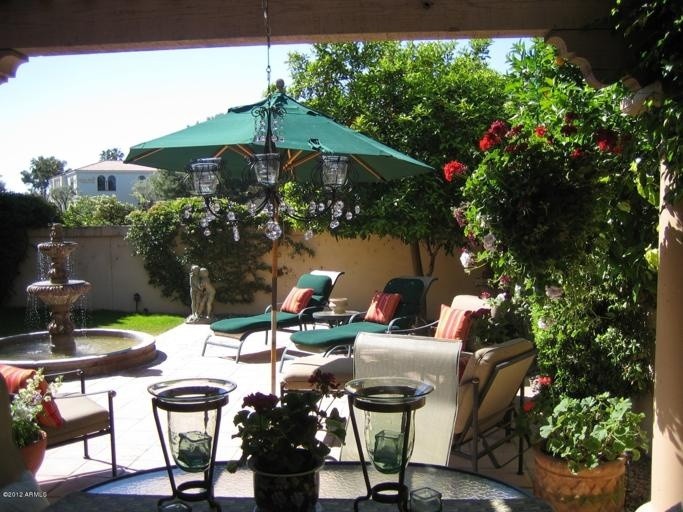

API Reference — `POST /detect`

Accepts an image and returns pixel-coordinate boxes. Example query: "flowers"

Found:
[5,368,66,446]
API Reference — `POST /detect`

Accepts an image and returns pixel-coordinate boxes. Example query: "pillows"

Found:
[0,363,61,425]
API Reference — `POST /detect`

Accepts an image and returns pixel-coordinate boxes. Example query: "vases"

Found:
[20,431,47,478]
[248,459,325,511]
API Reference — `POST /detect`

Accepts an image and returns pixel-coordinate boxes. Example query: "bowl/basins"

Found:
[328,298,348,313]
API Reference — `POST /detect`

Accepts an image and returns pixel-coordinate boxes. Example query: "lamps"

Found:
[180,0,363,245]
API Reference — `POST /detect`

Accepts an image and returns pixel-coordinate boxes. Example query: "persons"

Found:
[188,264,199,316]
[197,267,216,318]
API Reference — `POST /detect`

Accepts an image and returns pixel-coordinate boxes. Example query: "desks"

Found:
[41,456,555,510]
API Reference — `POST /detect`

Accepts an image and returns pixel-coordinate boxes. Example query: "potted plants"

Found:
[528,391,652,511]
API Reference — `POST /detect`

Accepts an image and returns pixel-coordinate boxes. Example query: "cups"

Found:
[408,486,442,512]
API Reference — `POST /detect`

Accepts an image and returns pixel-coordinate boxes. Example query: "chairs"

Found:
[199,270,535,477]
[0,374,50,511]
[0,365,119,480]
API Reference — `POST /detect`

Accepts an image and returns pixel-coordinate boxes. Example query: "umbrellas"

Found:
[121,80,437,396]
